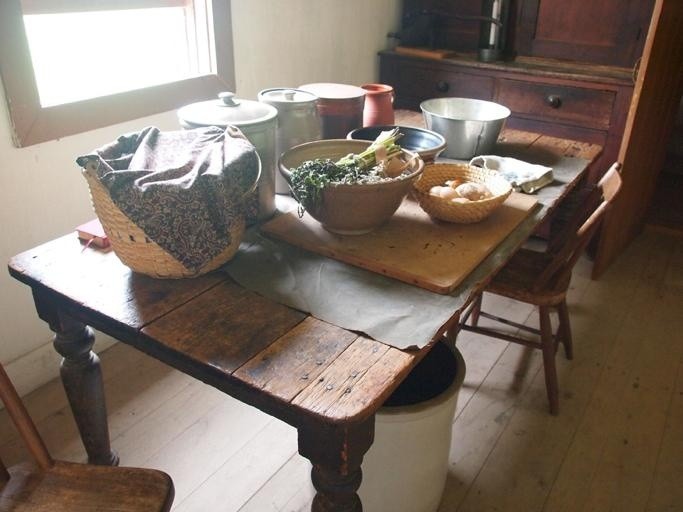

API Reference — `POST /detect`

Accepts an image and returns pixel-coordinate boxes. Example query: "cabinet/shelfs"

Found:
[378,2,679,280]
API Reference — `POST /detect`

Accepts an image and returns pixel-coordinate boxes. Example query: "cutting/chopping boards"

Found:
[257,162,540,294]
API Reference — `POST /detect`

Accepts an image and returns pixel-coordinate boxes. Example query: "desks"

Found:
[9,106,604,511]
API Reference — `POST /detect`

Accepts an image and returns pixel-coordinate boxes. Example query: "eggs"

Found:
[430,184,479,203]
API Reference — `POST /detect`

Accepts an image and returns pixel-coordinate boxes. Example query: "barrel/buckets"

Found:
[177,100,277,226]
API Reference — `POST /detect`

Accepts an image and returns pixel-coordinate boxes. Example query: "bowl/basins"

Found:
[347,124,446,167]
[420,96,512,162]
[277,139,423,236]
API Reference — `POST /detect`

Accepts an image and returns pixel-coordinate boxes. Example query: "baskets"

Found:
[80,150,262,279]
[409,161,513,224]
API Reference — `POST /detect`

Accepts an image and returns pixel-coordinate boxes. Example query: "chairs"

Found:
[1,361,175,512]
[436,161,628,417]
